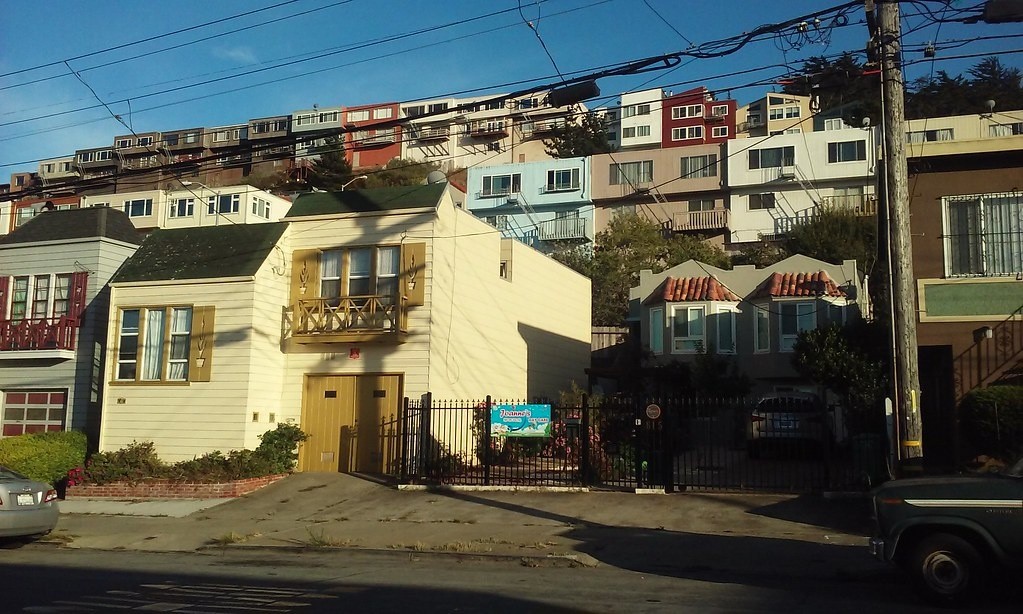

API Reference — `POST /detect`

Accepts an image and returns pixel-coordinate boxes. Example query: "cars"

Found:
[749,391,835,460]
[0,465,59,550]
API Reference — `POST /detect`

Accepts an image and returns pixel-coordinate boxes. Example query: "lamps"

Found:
[983,326,993,339]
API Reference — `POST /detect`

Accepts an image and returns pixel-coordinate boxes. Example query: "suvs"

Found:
[874,454,1023,611]
